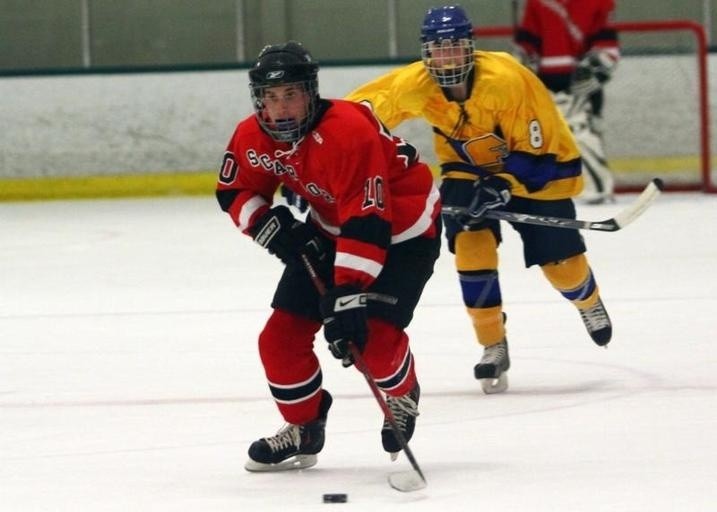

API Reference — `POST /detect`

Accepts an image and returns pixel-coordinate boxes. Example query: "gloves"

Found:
[570,48,620,98]
[250,205,328,273]
[461,175,511,224]
[324,290,367,368]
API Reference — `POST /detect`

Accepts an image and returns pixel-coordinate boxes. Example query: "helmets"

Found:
[248,41,318,89]
[420,5,473,44]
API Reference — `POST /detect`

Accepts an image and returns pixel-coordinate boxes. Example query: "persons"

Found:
[215,39,442,463]
[336,3,611,379]
[511,0,621,208]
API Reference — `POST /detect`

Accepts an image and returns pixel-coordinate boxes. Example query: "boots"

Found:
[577,295,612,346]
[248,421,325,464]
[382,383,420,453]
[474,336,509,379]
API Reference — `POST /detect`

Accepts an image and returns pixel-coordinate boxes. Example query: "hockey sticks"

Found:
[301,250,427,491]
[441,178,664,231]
